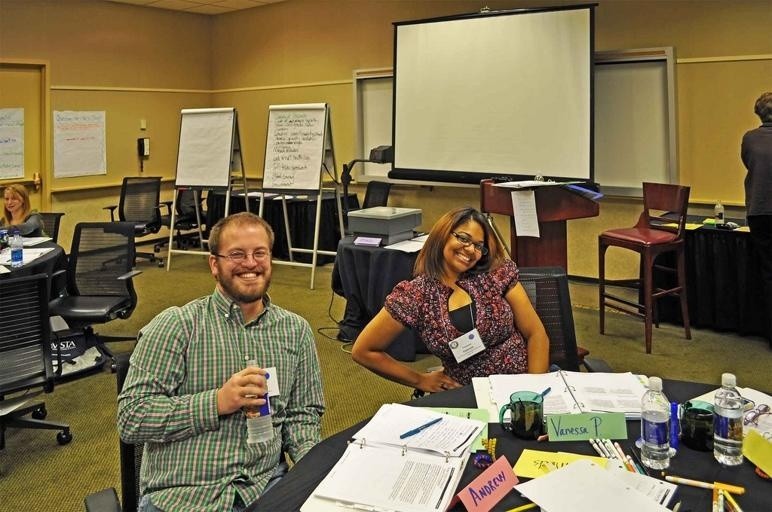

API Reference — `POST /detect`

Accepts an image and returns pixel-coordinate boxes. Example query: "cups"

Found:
[498,390,544,441]
[678,398,713,451]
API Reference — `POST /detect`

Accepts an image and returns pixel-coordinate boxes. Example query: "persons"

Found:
[741,92,772,348]
[116,212,326,512]
[0,184,51,237]
[351,207,550,393]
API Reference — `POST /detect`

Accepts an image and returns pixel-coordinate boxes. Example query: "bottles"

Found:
[715,199,725,228]
[534,171,544,185]
[1,230,24,267]
[640,376,670,469]
[712,372,744,466]
[668,402,679,451]
[245,359,276,445]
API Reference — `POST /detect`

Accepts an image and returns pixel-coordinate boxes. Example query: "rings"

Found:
[440,382,445,388]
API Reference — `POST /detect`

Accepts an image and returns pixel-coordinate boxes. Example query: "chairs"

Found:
[40,212,67,243]
[48,220,142,378]
[1,272,73,450]
[597,181,693,355]
[100,176,164,271]
[362,181,393,208]
[83,353,146,512]
[154,185,206,252]
[512,264,612,373]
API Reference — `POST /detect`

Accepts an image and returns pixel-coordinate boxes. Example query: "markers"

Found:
[178,186,192,191]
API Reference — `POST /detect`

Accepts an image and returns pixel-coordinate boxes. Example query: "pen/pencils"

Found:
[537,434,549,442]
[505,502,537,512]
[589,438,652,476]
[400,417,442,439]
[533,387,551,400]
[665,475,745,512]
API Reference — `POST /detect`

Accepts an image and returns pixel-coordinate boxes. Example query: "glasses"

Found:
[451,231,488,255]
[215,250,271,262]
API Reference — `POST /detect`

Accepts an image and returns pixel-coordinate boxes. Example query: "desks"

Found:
[238,371,771,511]
[638,212,771,340]
[329,231,433,362]
[207,188,360,266]
[0,233,68,294]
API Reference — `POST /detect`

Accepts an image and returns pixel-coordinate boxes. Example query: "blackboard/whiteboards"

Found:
[174,107,237,191]
[261,103,329,196]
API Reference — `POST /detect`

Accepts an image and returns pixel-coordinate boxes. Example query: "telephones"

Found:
[137,138,149,156]
